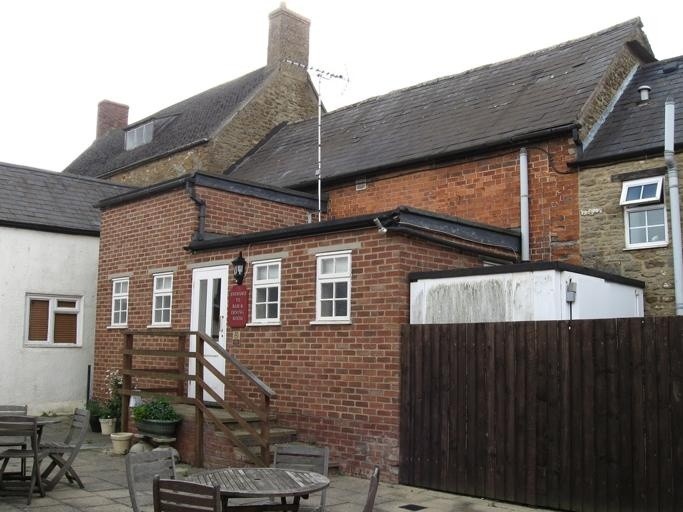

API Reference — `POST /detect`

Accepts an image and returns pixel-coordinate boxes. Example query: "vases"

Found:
[110,431,133,455]
[129,389,142,407]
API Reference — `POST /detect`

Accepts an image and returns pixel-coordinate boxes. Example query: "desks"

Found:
[0,414,68,497]
[183,467,331,512]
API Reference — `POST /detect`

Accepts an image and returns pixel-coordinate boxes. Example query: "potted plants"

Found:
[132,398,183,434]
[85,397,119,435]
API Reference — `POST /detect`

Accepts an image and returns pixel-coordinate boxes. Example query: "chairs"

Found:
[40,408,90,492]
[124,448,221,511]
[0,404,46,505]
[274,444,381,512]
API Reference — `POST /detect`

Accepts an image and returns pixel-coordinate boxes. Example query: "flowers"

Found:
[103,368,139,403]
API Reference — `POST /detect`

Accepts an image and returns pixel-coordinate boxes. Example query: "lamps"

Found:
[231,250,245,285]
